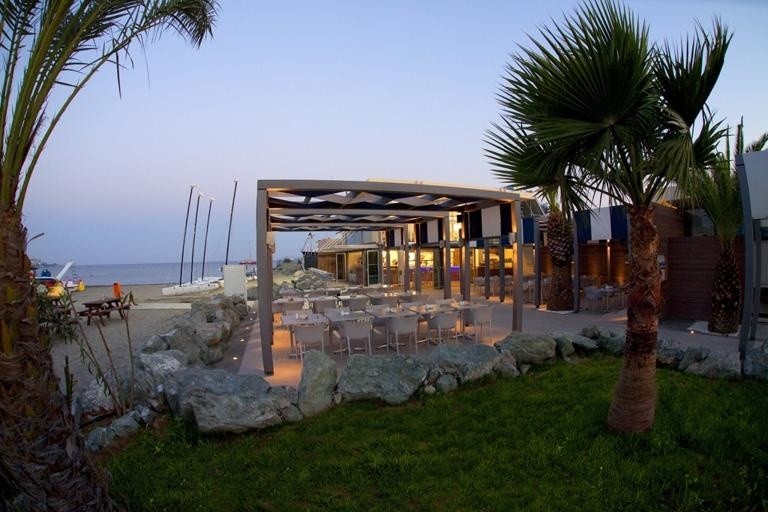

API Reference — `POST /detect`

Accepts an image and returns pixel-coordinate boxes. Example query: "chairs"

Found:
[274,282,494,366]
[471,274,626,316]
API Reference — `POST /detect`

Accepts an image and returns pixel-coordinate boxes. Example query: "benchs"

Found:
[76,298,132,326]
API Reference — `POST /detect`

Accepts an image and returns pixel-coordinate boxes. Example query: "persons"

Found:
[77,278,86,297]
